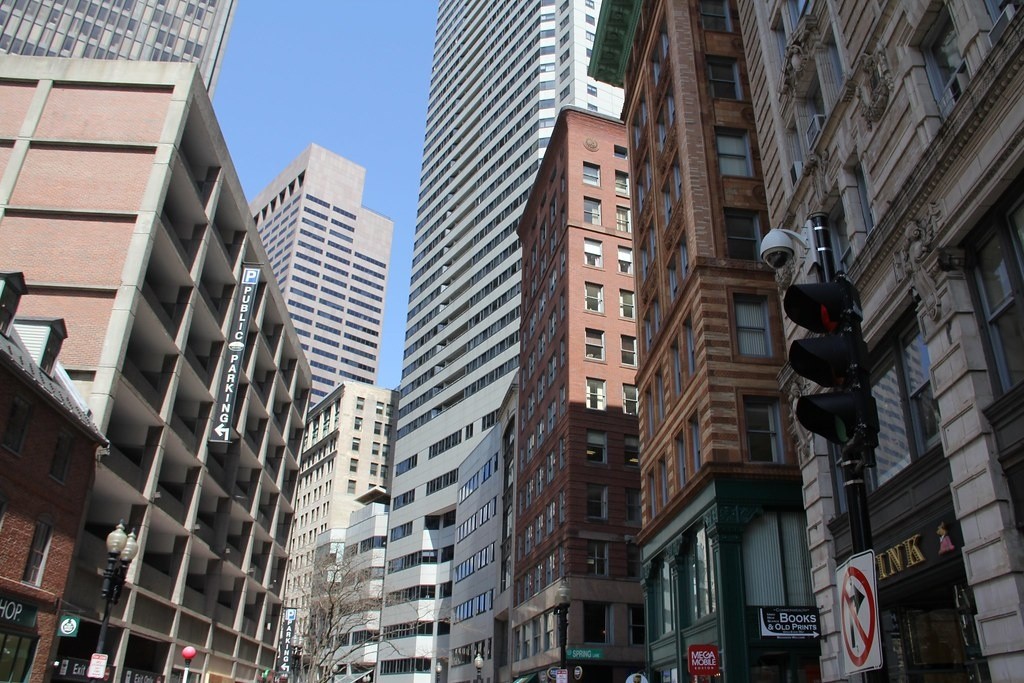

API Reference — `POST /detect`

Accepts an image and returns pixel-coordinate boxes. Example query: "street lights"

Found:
[434,662,443,683]
[290,633,305,683]
[474,653,484,683]
[87,517,141,683]
[554,577,573,683]
[331,664,338,683]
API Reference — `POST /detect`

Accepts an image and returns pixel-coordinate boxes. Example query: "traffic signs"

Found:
[759,606,822,639]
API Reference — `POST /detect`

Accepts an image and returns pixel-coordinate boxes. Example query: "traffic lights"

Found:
[783,278,880,451]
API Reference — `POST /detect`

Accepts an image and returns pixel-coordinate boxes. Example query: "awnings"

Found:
[513,672,538,683]
[336,669,373,683]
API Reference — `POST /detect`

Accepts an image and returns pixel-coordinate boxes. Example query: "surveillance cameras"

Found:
[759,231,795,269]
